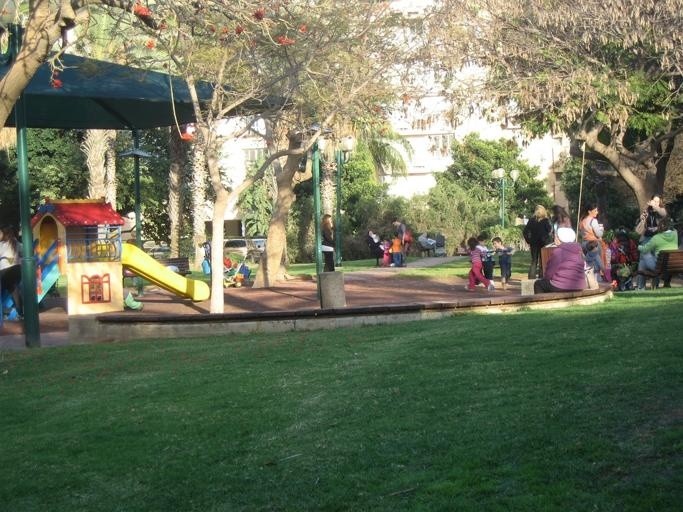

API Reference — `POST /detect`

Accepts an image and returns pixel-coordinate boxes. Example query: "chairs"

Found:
[416,241,434,256]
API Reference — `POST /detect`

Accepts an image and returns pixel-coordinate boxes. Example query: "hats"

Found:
[557,227,576,243]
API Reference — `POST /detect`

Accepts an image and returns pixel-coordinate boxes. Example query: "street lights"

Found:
[1,1,41,346]
[312,134,354,299]
[492,168,520,228]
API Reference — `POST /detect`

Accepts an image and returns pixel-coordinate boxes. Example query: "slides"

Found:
[122,243,210,302]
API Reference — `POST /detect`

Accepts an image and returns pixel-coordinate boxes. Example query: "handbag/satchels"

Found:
[584,267,599,290]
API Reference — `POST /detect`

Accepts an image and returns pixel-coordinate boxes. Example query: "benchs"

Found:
[123,257,192,288]
[636,250,683,287]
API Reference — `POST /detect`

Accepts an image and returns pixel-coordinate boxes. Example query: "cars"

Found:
[223,238,265,258]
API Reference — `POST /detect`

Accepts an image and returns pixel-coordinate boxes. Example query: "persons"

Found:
[123,288,144,311]
[366,220,408,267]
[0,208,25,323]
[321,214,336,272]
[464,235,516,292]
[419,232,436,256]
[523,193,679,295]
